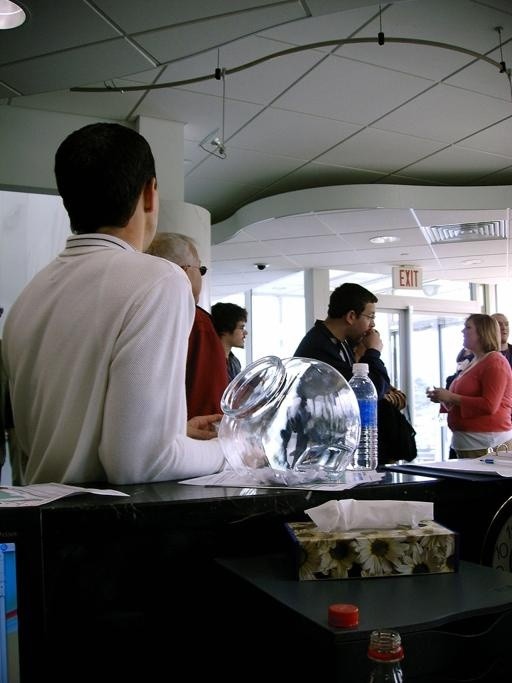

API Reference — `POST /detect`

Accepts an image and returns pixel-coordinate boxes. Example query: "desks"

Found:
[1,466,512,683]
[215,568,512,683]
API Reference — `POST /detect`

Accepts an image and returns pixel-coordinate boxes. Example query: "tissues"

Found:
[285,499,456,581]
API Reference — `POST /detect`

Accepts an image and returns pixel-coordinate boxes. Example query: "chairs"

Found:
[483,496,512,571]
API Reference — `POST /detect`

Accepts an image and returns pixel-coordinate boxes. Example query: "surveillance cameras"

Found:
[257,262,265,270]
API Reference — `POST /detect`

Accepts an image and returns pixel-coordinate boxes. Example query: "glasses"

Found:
[361,313,376,320]
[181,264,207,276]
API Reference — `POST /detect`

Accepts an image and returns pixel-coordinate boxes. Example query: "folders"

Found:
[378,444,512,482]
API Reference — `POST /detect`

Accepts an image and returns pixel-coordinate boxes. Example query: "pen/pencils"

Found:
[479,458,512,467]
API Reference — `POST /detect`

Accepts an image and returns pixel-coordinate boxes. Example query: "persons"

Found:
[146,231,226,440]
[430,318,512,457]
[0,124,232,485]
[445,312,511,407]
[207,304,248,395]
[296,285,405,460]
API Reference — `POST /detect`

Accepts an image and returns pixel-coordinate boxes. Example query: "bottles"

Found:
[348,364,378,470]
[360,632,414,683]
